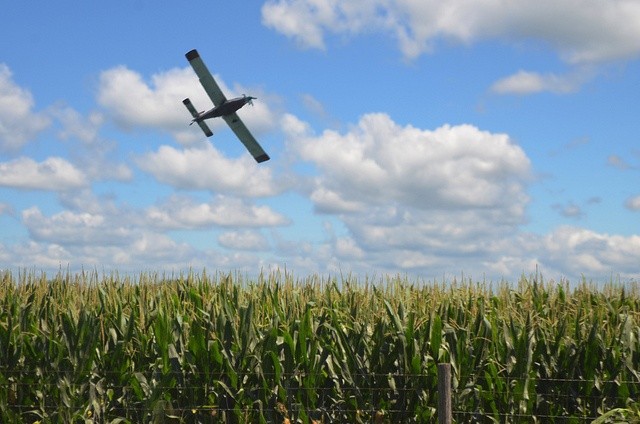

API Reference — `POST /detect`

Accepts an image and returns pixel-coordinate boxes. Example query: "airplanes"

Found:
[182,49,270,163]
